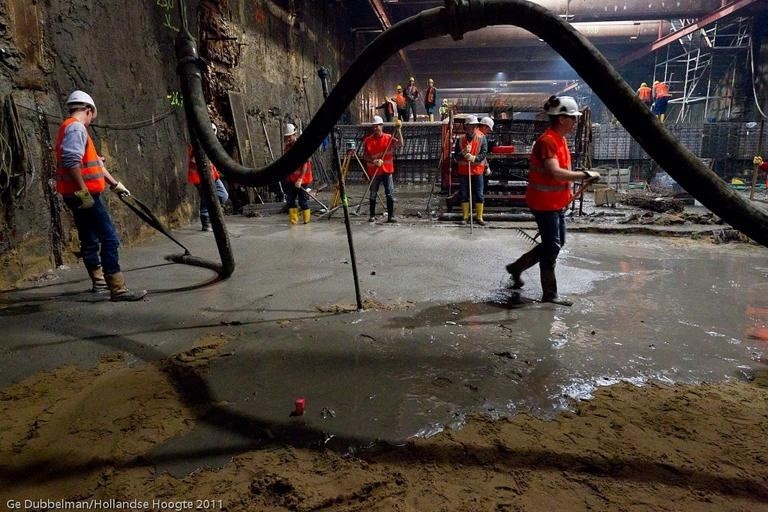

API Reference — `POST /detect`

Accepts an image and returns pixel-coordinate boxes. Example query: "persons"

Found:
[446,117,496,212]
[637,82,651,107]
[754,156,768,193]
[375,76,438,121]
[283,124,313,225]
[455,115,488,226]
[506,97,601,305]
[651,80,671,123]
[55,90,147,302]
[363,116,403,222]
[189,122,229,231]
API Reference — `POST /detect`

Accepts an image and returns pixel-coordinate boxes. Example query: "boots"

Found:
[660,114,665,124]
[289,208,299,225]
[475,203,486,226]
[540,270,573,305]
[88,266,110,295]
[462,203,471,222]
[200,216,213,232]
[387,200,397,222]
[104,272,147,301]
[429,113,434,123]
[369,199,376,222]
[505,253,537,288]
[303,208,311,224]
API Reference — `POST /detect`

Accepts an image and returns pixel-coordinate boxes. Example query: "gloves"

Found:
[374,159,382,166]
[583,171,601,183]
[295,178,302,187]
[74,190,95,209]
[110,182,131,199]
[466,156,475,161]
[393,119,402,127]
[461,145,471,154]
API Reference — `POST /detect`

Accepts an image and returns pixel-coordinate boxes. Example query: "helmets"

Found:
[427,78,434,83]
[543,95,583,116]
[384,96,389,100]
[641,83,647,87]
[397,85,402,89]
[464,116,479,124]
[284,124,296,136]
[654,81,660,84]
[371,116,384,124]
[408,77,415,81]
[66,90,97,120]
[480,117,495,132]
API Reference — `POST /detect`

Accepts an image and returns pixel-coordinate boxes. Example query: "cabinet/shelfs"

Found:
[441,103,590,219]
